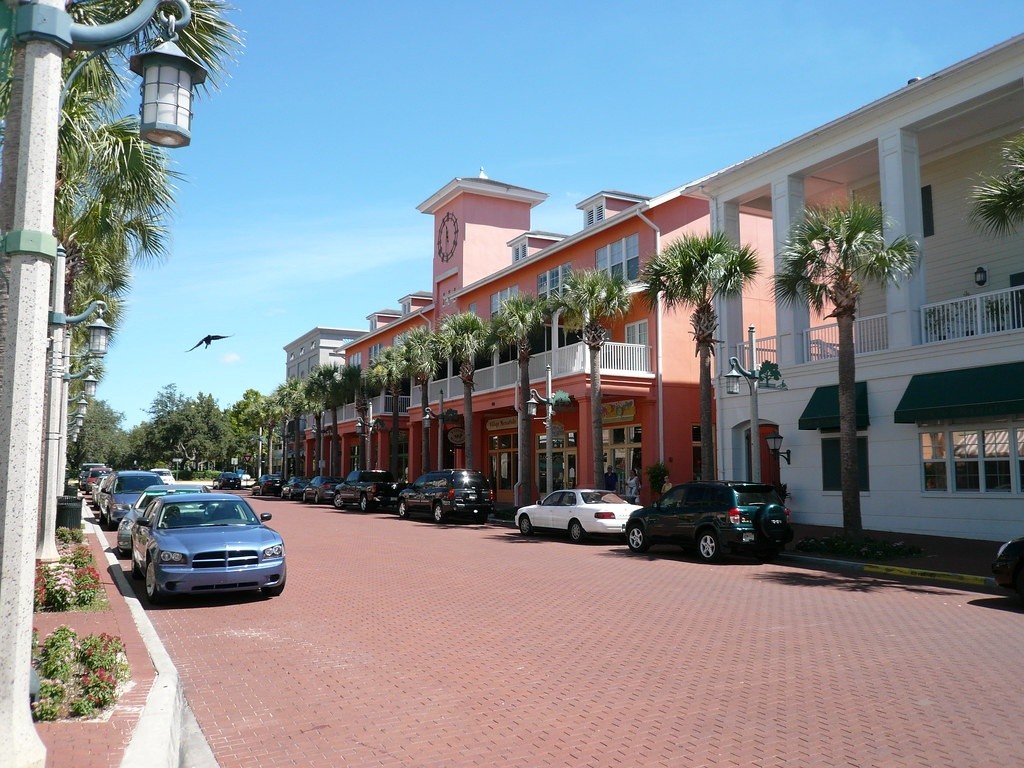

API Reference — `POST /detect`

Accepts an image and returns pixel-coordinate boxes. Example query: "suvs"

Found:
[624,480,792,564]
[395,469,495,524]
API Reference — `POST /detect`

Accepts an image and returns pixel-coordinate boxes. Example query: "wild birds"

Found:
[184,333,236,353]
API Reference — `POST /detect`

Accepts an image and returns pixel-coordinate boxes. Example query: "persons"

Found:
[626,470,641,505]
[661,475,672,505]
[604,465,618,491]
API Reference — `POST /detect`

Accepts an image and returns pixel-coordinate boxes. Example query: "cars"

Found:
[301,475,345,505]
[150,469,177,492]
[992,537,1023,601]
[251,474,288,496]
[99,471,169,531]
[280,476,311,501]
[515,489,644,545]
[129,493,287,606]
[213,472,241,490]
[117,484,211,558]
[78,462,112,511]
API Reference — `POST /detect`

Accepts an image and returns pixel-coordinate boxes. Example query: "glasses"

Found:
[664,477,668,479]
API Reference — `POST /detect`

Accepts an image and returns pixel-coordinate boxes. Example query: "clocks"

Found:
[765,430,791,465]
[436,211,459,263]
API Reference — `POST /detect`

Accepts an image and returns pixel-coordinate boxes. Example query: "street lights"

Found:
[276,388,444,481]
[0,1,208,768]
[56,330,99,497]
[526,364,553,503]
[35,243,112,570]
[724,324,762,484]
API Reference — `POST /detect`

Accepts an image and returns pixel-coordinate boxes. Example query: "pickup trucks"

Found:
[333,470,414,514]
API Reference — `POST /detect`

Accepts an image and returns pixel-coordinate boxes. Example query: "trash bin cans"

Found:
[57,496,83,531]
[64,485,78,496]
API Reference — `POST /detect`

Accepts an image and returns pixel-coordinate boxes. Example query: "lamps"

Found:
[974,266,987,286]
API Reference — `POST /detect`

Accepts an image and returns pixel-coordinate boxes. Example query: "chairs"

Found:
[164,506,180,518]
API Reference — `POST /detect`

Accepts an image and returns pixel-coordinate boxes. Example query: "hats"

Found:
[608,465,612,469]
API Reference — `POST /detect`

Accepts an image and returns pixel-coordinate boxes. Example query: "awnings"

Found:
[894,362,1024,424]
[799,381,870,430]
